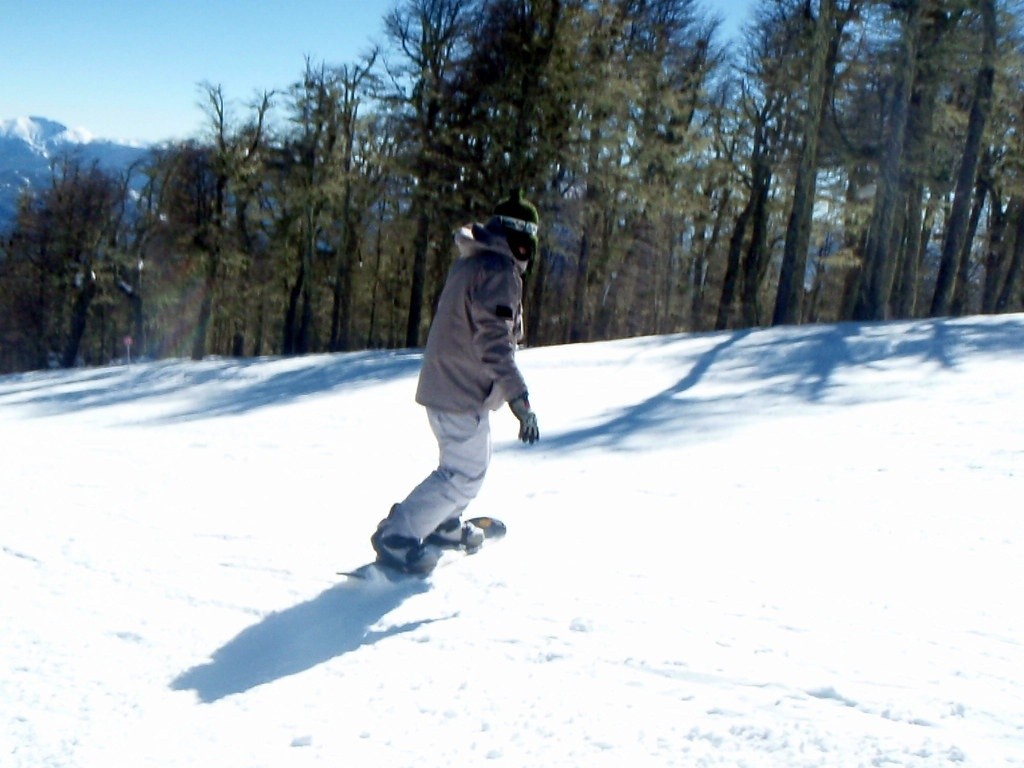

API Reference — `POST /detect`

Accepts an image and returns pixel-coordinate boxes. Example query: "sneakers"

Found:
[373,526,439,573]
[430,515,486,547]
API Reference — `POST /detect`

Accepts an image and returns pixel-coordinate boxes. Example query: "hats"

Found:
[493,185,539,275]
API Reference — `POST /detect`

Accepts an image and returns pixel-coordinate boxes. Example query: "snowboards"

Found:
[337,515,507,580]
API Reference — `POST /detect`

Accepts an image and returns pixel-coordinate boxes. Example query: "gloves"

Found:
[507,390,540,445]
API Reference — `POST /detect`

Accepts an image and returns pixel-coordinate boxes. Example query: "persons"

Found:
[370,199,540,576]
